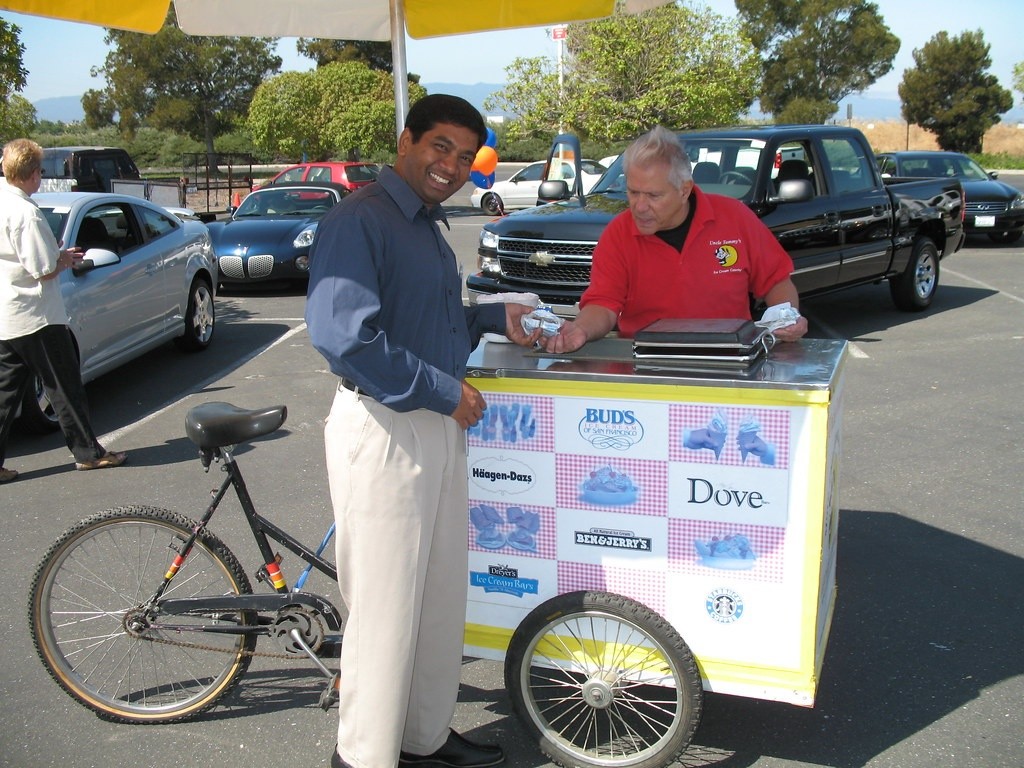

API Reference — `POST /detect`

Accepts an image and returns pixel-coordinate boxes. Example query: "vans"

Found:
[0,145,141,194]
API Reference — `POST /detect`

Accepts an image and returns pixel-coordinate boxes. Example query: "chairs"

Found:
[912,167,931,177]
[57,217,110,256]
[773,159,854,196]
[691,162,757,201]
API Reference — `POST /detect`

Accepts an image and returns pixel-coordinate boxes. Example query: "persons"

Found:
[306,93,542,768]
[539,129,807,354]
[0,138,128,482]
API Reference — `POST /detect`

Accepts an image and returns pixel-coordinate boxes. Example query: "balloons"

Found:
[472,146,497,176]
[471,171,495,189]
[485,127,495,148]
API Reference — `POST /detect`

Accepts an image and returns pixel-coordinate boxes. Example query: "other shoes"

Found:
[0,467,19,482]
[76,451,130,470]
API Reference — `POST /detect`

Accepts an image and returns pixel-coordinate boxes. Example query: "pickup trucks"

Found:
[465,122,965,320]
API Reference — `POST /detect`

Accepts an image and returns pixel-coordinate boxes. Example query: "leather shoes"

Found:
[399,726,506,768]
[330,743,348,768]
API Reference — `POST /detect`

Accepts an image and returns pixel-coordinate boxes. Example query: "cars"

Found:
[469,156,631,216]
[852,151,1024,245]
[251,161,380,200]
[203,181,357,295]
[19,191,219,437]
[597,138,805,179]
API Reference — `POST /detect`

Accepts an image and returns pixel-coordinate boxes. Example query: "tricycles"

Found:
[28,317,851,768]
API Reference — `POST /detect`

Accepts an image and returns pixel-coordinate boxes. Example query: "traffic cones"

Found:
[231,192,242,209]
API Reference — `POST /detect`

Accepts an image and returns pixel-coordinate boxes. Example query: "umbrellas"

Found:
[0,1,676,146]
